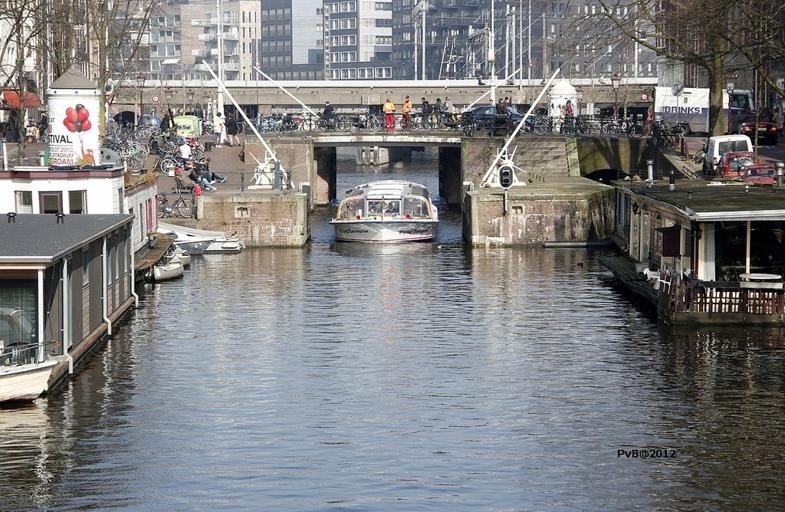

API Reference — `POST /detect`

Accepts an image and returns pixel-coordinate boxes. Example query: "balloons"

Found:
[63,104,91,133]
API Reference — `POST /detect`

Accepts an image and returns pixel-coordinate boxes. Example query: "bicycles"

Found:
[102,122,207,177]
[156,184,195,219]
[236,112,679,156]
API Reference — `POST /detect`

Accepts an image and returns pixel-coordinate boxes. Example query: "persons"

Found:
[435,98,445,129]
[504,97,511,108]
[566,100,573,134]
[75,145,95,170]
[560,105,568,135]
[401,95,412,132]
[157,132,227,192]
[36,111,48,140]
[489,100,496,113]
[383,98,397,133]
[420,97,433,130]
[498,98,507,114]
[321,102,334,124]
[223,114,241,147]
[212,112,224,149]
[443,96,456,126]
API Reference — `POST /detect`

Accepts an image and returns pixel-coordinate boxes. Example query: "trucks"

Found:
[654,85,764,137]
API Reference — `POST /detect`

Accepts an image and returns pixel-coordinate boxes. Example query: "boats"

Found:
[0,211,138,409]
[326,238,435,259]
[327,179,439,244]
[595,177,785,329]
[144,220,245,281]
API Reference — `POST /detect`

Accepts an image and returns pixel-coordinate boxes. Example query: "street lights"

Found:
[136,71,146,125]
[203,91,210,122]
[725,71,738,148]
[611,71,621,121]
[185,87,195,115]
[165,86,174,112]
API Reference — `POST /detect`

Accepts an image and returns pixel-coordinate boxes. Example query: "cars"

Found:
[462,105,534,130]
[701,113,785,188]
